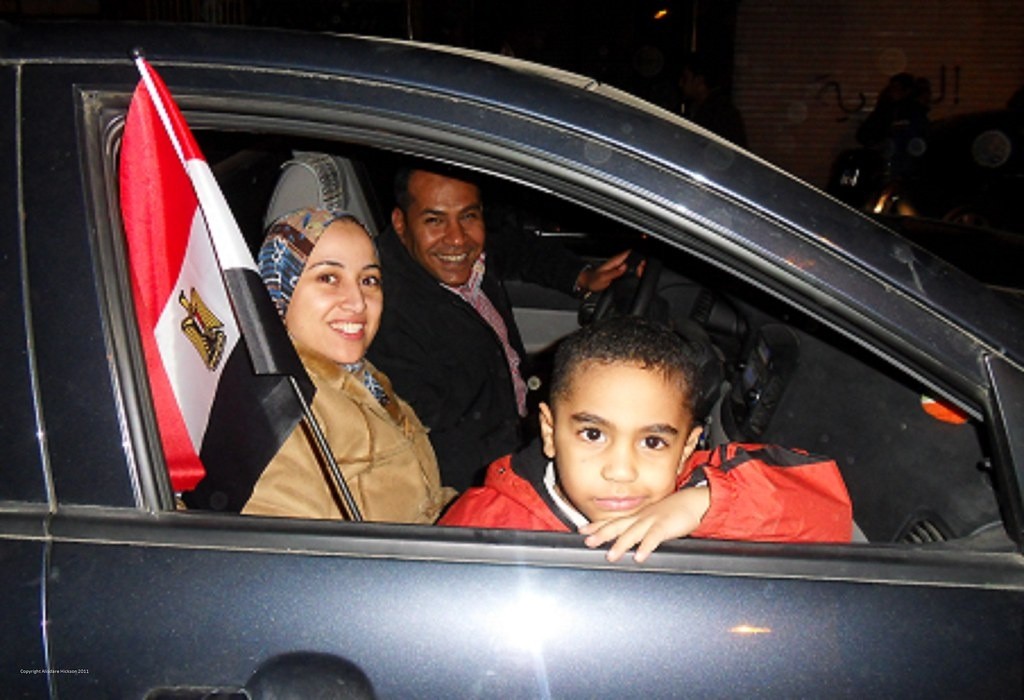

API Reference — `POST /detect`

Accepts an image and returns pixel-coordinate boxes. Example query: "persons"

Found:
[237,203,456,527]
[876,71,932,125]
[681,51,746,150]
[429,310,856,564]
[365,165,646,495]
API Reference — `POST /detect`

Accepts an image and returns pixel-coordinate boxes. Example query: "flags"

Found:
[119,57,316,517]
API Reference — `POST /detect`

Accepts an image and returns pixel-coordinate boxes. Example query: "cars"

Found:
[0,11,1023,700]
